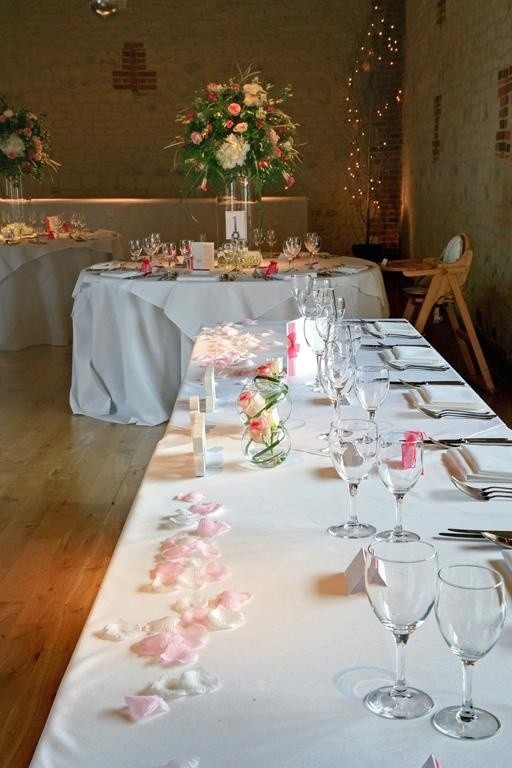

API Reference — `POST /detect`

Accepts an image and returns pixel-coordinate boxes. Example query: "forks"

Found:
[450,476,512,502]
[367,331,417,339]
[399,379,466,388]
[375,341,431,348]
[420,407,496,420]
[428,435,511,447]
[385,361,449,372]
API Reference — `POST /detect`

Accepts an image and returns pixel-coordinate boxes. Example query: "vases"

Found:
[212,173,261,251]
[239,422,292,469]
[5,171,25,224]
[351,244,401,293]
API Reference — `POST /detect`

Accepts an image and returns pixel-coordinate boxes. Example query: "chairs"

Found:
[381,231,497,391]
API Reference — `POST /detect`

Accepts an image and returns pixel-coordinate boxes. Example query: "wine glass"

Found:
[375,430,424,544]
[357,366,392,447]
[327,419,377,537]
[251,228,321,274]
[78,213,87,238]
[128,233,195,280]
[362,541,439,721]
[295,279,358,456]
[436,569,508,741]
[221,238,248,278]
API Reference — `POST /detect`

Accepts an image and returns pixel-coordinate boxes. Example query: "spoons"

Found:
[481,531,512,550]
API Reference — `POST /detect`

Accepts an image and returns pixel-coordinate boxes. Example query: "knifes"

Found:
[387,381,461,384]
[439,528,511,539]
[361,344,429,348]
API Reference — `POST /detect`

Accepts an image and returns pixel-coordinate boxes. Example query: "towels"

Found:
[378,345,441,366]
[176,269,225,281]
[402,385,482,411]
[364,321,421,340]
[333,258,368,273]
[502,549,512,576]
[443,443,512,484]
[98,269,147,279]
[274,269,316,280]
[90,260,122,271]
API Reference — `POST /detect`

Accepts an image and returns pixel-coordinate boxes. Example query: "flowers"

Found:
[0,100,65,190]
[236,355,284,445]
[163,62,306,229]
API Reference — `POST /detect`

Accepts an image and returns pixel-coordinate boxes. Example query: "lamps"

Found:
[89,0,120,20]
